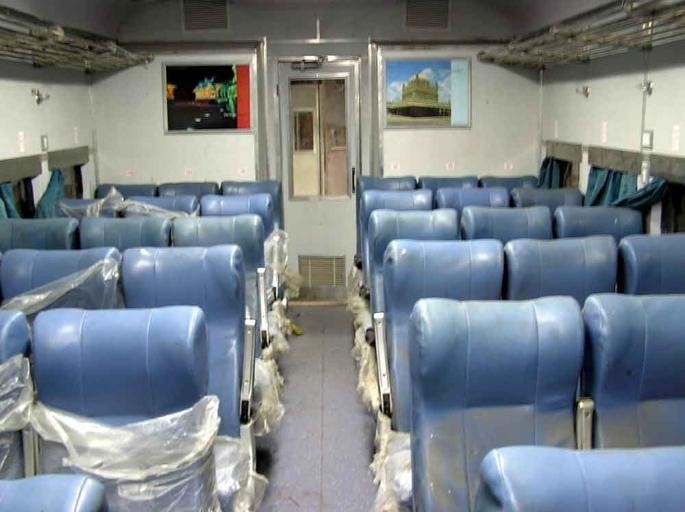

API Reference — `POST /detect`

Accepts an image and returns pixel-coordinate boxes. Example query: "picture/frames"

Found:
[379,51,476,130]
[160,53,258,135]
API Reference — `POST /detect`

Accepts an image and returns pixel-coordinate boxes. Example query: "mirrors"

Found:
[289,74,353,201]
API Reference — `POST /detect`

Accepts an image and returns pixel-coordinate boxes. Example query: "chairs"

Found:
[0,179,283,510]
[359,175,684,510]
